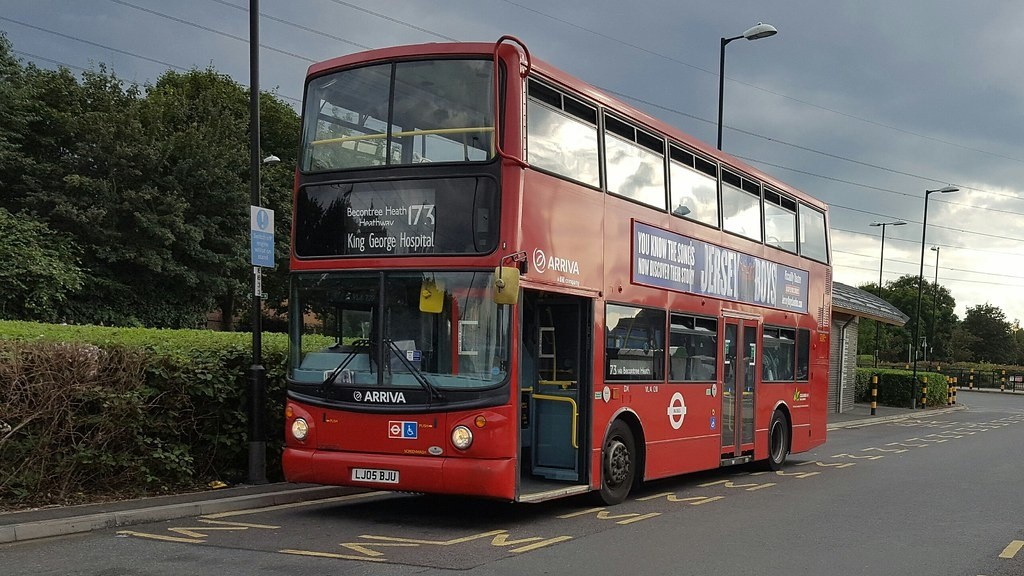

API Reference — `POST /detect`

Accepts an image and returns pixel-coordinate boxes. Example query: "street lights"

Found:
[911,185,959,410]
[869,220,908,367]
[717,21,778,150]
[930,246,940,364]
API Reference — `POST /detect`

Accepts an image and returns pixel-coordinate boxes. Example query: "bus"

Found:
[281,34,834,507]
